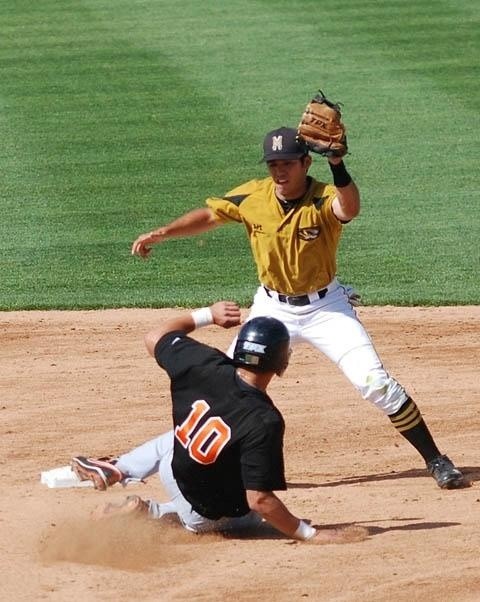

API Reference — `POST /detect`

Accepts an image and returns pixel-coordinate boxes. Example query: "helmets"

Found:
[233,316,290,378]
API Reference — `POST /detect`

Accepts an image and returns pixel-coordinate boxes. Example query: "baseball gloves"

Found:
[296,93,348,159]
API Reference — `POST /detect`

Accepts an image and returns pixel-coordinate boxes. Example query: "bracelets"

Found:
[192,306,213,330]
[328,161,352,190]
[293,518,317,541]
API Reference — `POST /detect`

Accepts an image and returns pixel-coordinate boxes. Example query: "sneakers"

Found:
[426,455,465,490]
[90,495,149,522]
[71,455,124,491]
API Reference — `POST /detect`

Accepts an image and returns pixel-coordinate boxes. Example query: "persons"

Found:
[69,301,350,544]
[131,127,464,489]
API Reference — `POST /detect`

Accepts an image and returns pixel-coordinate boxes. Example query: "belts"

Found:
[263,286,328,306]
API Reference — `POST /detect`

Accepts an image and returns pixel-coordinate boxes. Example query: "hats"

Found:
[259,127,309,163]
